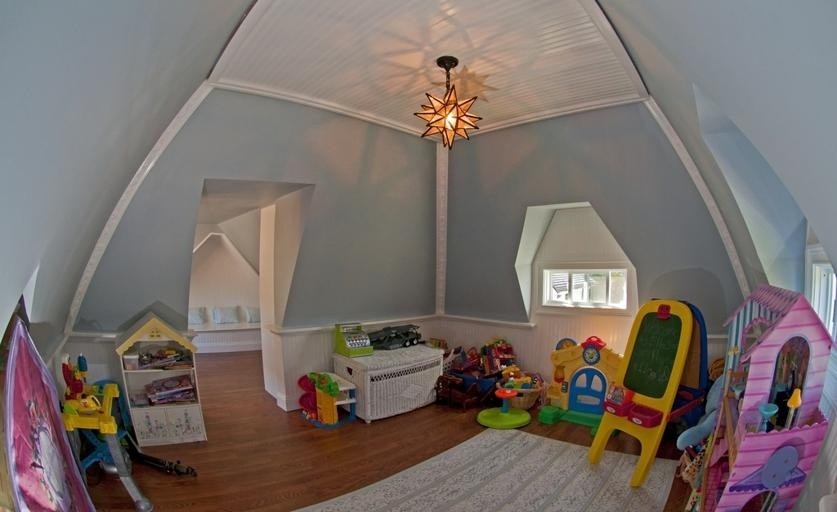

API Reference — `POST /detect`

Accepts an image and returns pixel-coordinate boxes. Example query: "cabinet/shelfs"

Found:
[116,310,207,448]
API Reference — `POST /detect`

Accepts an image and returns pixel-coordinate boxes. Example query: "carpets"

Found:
[292,426,678,512]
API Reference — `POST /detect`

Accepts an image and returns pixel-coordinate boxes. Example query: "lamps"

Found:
[414,56,483,150]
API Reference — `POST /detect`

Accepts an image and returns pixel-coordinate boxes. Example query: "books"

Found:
[136,353,193,370]
[127,374,196,406]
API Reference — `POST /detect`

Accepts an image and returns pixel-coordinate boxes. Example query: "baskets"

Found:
[496,379,544,410]
